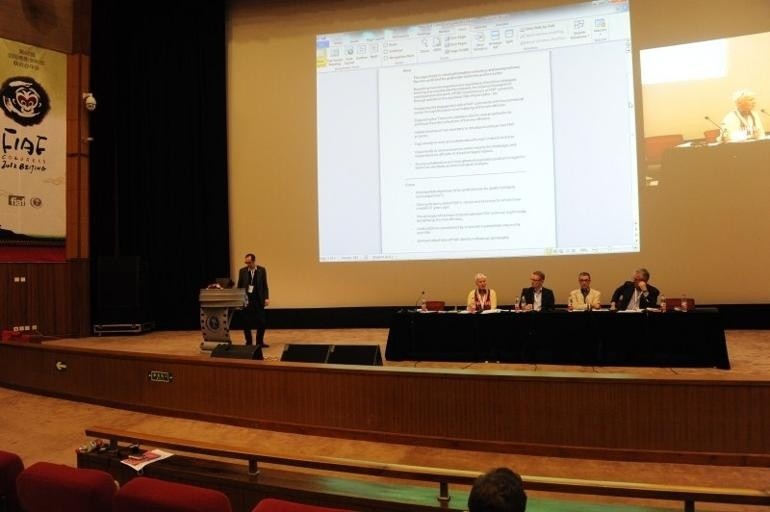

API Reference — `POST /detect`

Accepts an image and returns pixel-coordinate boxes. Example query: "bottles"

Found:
[514,295,520,310]
[521,296,527,310]
[421,297,427,312]
[568,295,573,312]
[660,294,666,313]
[681,293,688,312]
[719,123,726,136]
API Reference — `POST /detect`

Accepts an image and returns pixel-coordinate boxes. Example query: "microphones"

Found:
[704,116,725,143]
[416,291,425,312]
[761,109,769,122]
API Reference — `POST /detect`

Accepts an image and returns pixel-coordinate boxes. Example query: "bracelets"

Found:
[643,289,648,293]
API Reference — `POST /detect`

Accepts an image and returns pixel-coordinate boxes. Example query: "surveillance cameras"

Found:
[83,92,96,110]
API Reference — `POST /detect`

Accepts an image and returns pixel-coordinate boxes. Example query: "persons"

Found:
[464,464,530,512]
[608,268,661,313]
[715,90,766,143]
[565,271,602,312]
[517,270,556,312]
[466,271,499,313]
[236,251,271,348]
